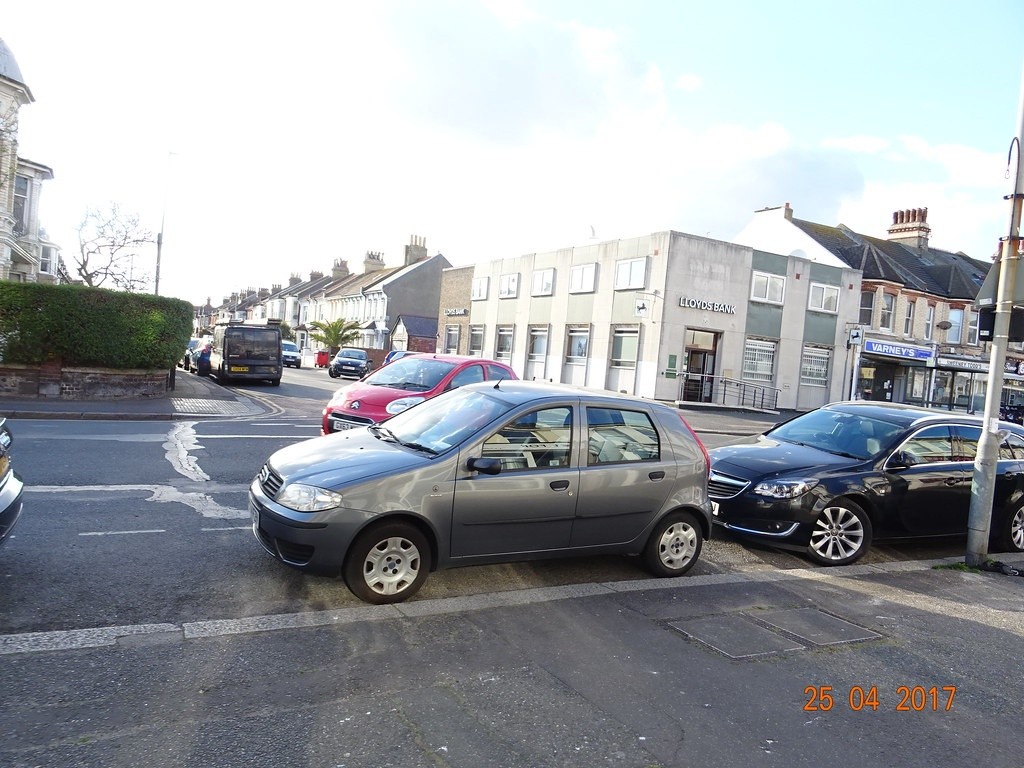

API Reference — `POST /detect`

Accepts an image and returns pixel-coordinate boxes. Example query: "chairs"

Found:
[505,414,537,445]
[535,413,570,466]
[848,422,874,456]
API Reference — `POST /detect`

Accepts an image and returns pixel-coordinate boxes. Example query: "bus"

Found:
[210,319,284,386]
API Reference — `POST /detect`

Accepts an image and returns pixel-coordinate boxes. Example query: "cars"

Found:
[178,338,213,376]
[247,379,713,604]
[0,417,25,550]
[704,400,1024,566]
[320,350,538,442]
[329,347,372,381]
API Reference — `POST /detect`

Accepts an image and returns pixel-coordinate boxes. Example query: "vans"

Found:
[280,339,301,368]
